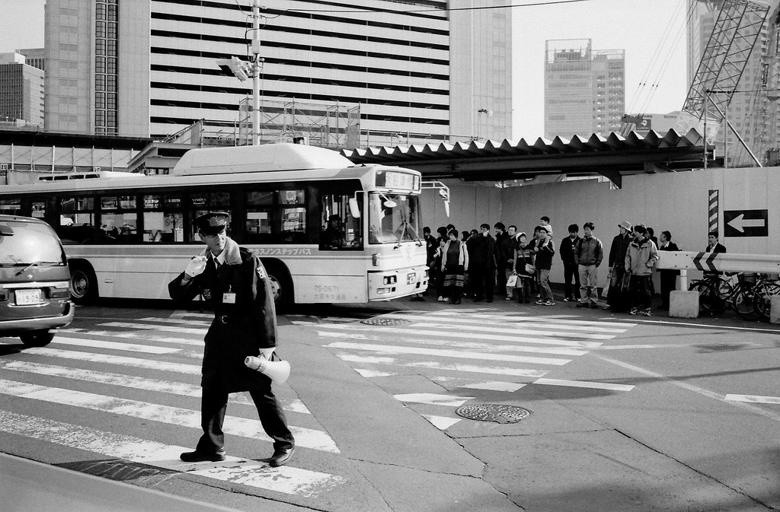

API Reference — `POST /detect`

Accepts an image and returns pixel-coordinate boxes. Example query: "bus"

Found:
[1,143,452,314]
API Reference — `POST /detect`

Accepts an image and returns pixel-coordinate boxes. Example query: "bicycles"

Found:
[688,267,779,322]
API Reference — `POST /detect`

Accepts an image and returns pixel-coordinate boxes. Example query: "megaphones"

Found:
[245,352,290,383]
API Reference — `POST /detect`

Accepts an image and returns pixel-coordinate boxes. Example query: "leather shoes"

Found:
[180,451,225,462]
[270,444,295,466]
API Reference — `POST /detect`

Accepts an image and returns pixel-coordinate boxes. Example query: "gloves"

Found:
[185,256,208,278]
[259,347,276,361]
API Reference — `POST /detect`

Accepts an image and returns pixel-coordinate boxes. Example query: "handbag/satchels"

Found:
[601,273,612,297]
[525,263,536,275]
[506,274,521,289]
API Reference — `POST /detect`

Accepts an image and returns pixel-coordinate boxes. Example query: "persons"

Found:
[705,233,726,253]
[168,211,295,467]
[423,215,679,317]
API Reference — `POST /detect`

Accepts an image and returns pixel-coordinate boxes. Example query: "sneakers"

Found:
[410,293,493,304]
[630,306,654,317]
[564,297,597,307]
[506,297,556,305]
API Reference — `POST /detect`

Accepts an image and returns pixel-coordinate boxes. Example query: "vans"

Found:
[0,213,75,348]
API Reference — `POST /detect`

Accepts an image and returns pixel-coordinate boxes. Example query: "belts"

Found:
[217,315,231,324]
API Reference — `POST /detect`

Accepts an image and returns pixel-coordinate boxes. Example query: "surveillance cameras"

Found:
[233,70,248,82]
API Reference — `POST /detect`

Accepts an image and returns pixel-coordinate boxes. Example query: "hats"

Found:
[618,221,632,232]
[516,232,527,242]
[192,212,229,235]
